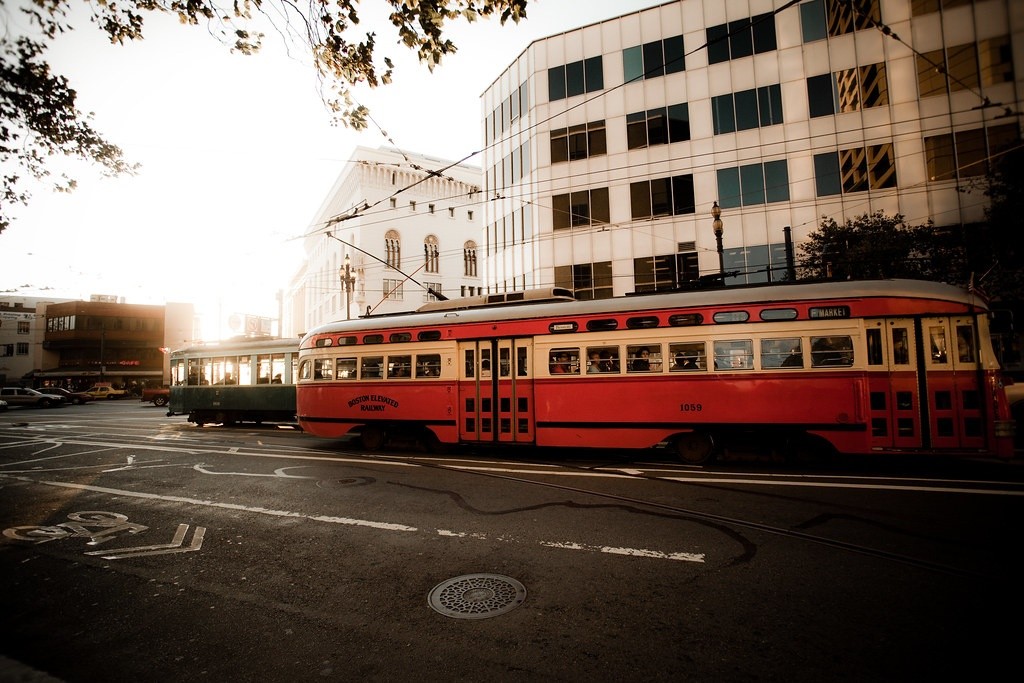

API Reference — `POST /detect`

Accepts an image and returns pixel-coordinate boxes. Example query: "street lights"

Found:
[712,200,725,288]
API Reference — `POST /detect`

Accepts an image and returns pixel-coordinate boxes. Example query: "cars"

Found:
[34,386,92,406]
[141,385,171,407]
[0,400,9,411]
[78,385,129,400]
[0,387,67,409]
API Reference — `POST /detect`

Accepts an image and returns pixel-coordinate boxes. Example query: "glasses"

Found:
[562,355,568,358]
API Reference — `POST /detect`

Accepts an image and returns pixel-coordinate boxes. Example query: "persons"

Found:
[66,378,146,393]
[183,349,702,385]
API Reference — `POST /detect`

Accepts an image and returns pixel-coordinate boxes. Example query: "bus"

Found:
[294,273,1017,466]
[166,334,302,427]
